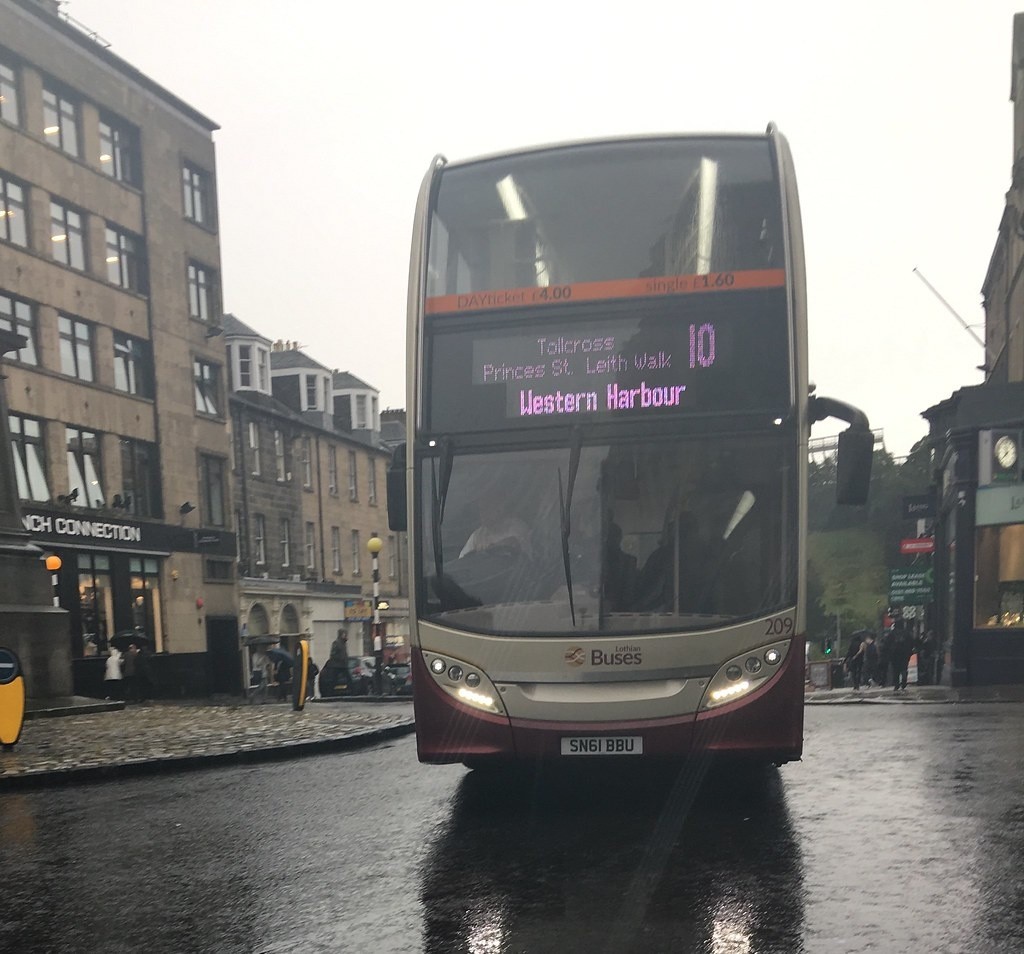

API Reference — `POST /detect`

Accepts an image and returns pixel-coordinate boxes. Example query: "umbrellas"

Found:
[267,646,294,666]
[242,634,280,645]
[108,630,150,645]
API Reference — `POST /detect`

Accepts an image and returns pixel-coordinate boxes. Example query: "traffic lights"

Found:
[824,636,833,656]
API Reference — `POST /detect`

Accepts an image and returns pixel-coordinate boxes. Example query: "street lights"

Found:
[364,536,384,695]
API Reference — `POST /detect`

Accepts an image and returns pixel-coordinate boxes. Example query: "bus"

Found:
[385,119,875,770]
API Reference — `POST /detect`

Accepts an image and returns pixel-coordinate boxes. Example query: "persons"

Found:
[251,627,356,696]
[844,633,863,691]
[103,643,154,704]
[454,493,537,577]
[881,617,914,691]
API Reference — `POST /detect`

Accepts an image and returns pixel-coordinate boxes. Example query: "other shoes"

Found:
[894,684,900,691]
[902,683,906,690]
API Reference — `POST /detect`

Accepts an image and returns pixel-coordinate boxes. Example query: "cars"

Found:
[318,656,413,696]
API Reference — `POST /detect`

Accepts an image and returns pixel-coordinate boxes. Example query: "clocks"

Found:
[994,436,1018,471]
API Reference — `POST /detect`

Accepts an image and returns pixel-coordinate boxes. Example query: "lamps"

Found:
[112,494,132,508]
[63,488,79,502]
[180,500,196,515]
